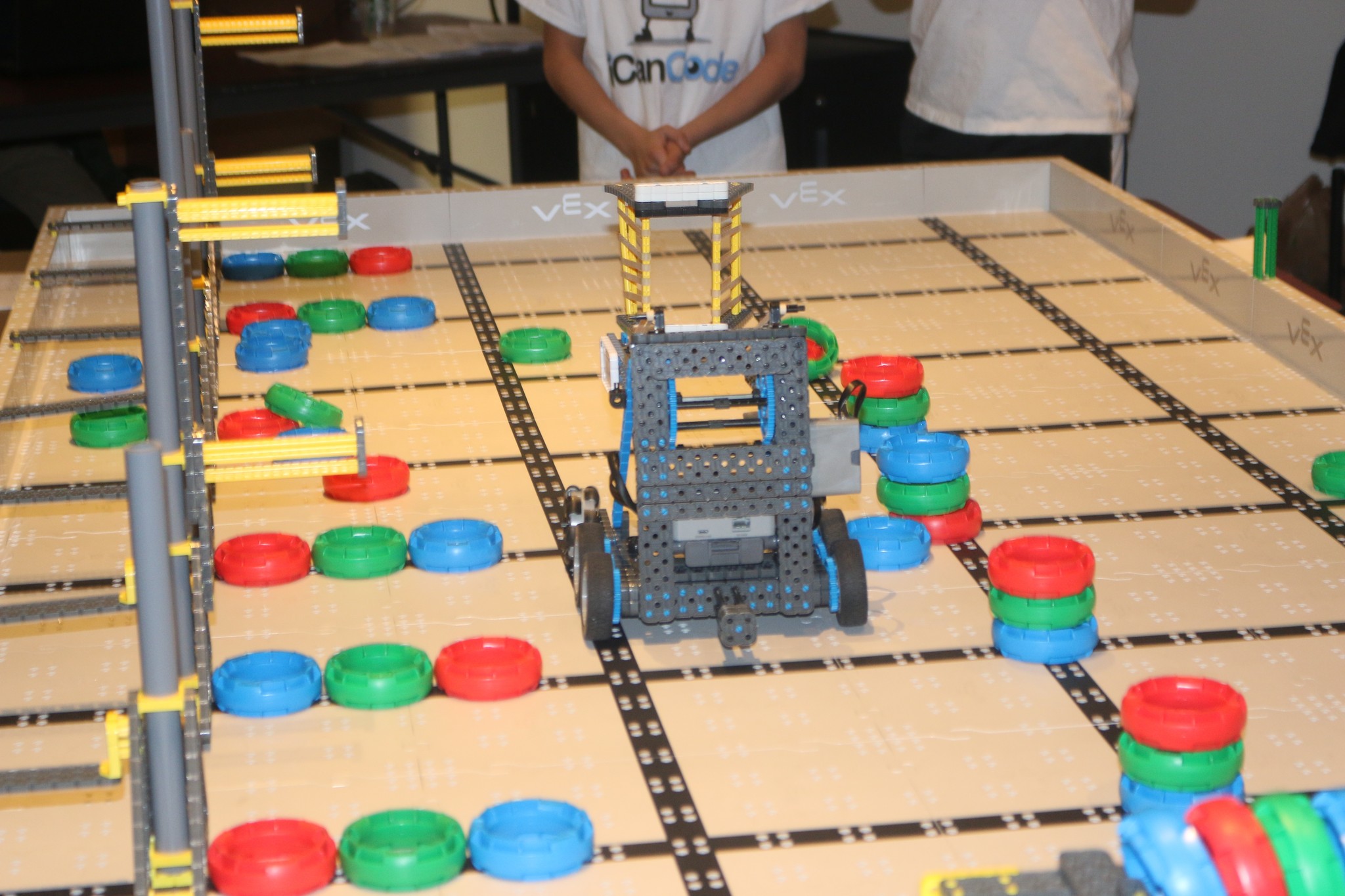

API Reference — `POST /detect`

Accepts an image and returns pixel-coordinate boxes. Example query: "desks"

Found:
[238,23,551,197]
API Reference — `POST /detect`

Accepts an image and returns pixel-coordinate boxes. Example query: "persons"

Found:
[517,0,832,182]
[870,0,1200,194]
